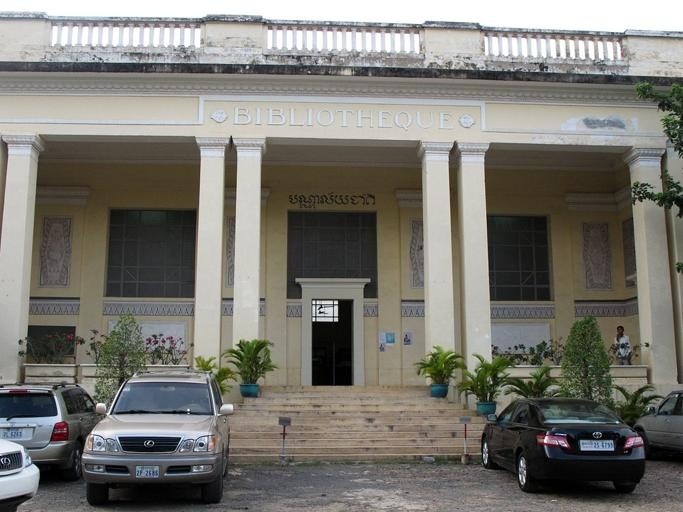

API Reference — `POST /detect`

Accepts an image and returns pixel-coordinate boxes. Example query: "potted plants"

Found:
[412,344,467,397]
[220,339,281,397]
[454,352,517,416]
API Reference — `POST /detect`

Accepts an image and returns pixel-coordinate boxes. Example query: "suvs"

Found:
[1,381,103,481]
[79,369,233,504]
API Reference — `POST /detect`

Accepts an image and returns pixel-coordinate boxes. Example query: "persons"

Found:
[613,325,633,366]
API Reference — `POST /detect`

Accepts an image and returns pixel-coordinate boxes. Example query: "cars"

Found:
[634,390,682,456]
[0,438,40,511]
[479,398,645,497]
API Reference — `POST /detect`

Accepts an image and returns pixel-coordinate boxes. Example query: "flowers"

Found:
[145,334,194,364]
[42,333,85,361]
